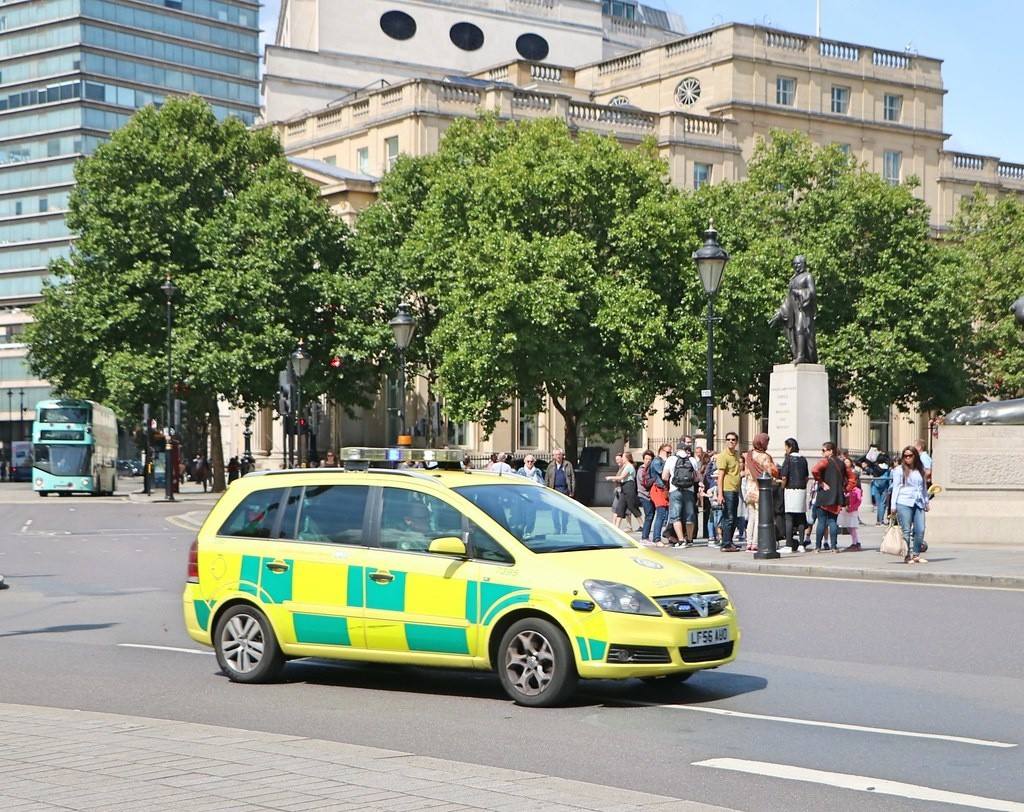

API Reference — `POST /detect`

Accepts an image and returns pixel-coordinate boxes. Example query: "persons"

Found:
[856,439,932,563]
[399,462,428,469]
[226,456,241,485]
[777,438,809,554]
[240,459,250,478]
[718,431,745,552]
[319,451,340,468]
[297,505,335,541]
[735,433,778,551]
[281,461,318,469]
[192,454,214,493]
[487,453,546,539]
[782,255,816,365]
[462,455,474,469]
[470,492,513,556]
[395,502,442,552]
[142,456,147,493]
[544,446,576,535]
[604,434,722,548]
[802,441,863,553]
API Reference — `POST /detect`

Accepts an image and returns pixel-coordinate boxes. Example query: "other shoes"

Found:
[562,523,566,534]
[815,549,820,552]
[624,527,632,533]
[635,526,645,532]
[803,540,812,548]
[721,545,739,552]
[709,540,722,547]
[746,546,758,553]
[641,538,655,545]
[776,546,791,552]
[904,556,915,564]
[798,546,805,552]
[832,547,839,552]
[653,541,666,547]
[912,555,928,564]
[555,528,561,535]
[846,542,860,551]
[671,539,694,548]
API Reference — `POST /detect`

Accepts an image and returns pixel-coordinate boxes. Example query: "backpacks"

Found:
[671,454,694,489]
[642,459,660,488]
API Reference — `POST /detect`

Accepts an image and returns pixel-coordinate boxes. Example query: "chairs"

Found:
[380,497,406,549]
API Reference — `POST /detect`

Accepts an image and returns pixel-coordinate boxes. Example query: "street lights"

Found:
[691,216,731,541]
[291,336,310,470]
[387,302,417,436]
[160,271,179,501]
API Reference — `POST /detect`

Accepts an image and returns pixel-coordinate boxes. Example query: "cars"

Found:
[117,459,144,477]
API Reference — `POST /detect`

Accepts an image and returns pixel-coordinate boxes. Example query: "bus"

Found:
[31,397,119,497]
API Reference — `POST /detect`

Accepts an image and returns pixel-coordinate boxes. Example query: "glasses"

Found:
[726,438,737,442]
[662,449,672,455]
[904,453,915,459]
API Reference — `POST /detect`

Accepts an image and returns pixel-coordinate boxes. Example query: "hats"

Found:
[497,453,507,461]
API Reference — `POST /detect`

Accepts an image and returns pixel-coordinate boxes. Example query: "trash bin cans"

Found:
[574,468,595,506]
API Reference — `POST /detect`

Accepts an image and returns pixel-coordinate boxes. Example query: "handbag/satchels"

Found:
[881,513,909,557]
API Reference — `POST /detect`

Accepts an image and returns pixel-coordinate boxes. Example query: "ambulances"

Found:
[181,458,740,708]
